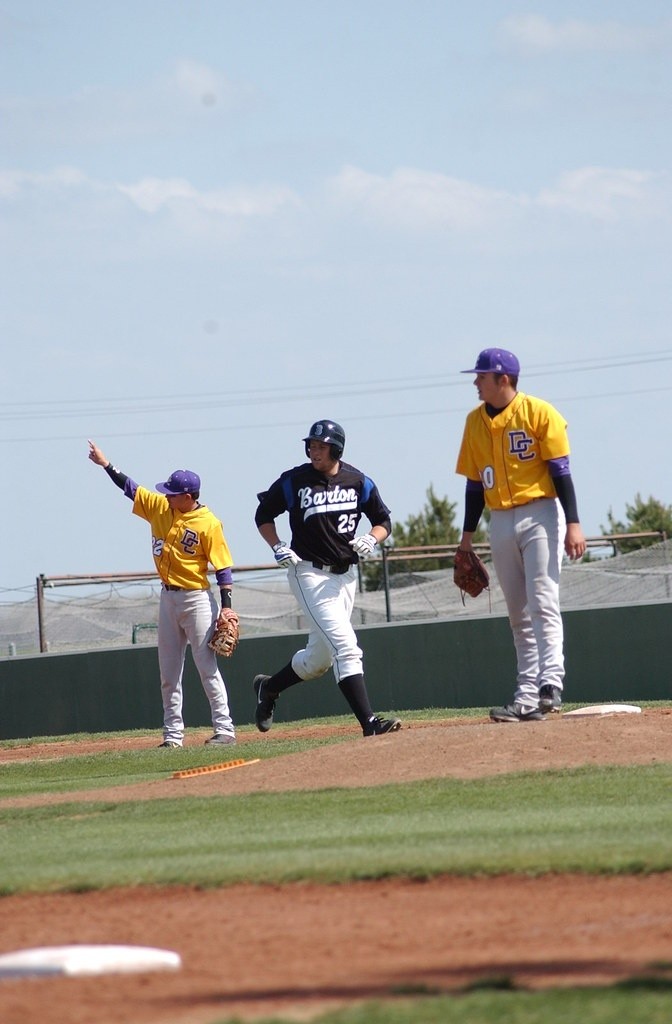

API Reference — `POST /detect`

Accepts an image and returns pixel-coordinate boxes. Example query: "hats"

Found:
[155,470,200,494]
[459,348,519,375]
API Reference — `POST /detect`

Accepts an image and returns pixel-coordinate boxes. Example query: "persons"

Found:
[254,419,401,738]
[453,348,586,718]
[87,440,237,748]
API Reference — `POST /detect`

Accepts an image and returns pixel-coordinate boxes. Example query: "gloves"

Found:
[272,541,302,568]
[348,534,378,560]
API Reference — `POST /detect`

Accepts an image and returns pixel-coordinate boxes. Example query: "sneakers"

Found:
[489,701,547,722]
[252,674,281,732]
[362,714,403,737]
[538,684,562,715]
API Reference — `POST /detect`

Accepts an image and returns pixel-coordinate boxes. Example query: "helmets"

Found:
[302,420,346,460]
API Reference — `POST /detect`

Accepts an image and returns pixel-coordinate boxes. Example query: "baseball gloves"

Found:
[453,546,492,614]
[206,608,240,658]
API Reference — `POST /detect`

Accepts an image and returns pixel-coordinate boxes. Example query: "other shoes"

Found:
[158,741,180,748]
[203,734,237,747]
